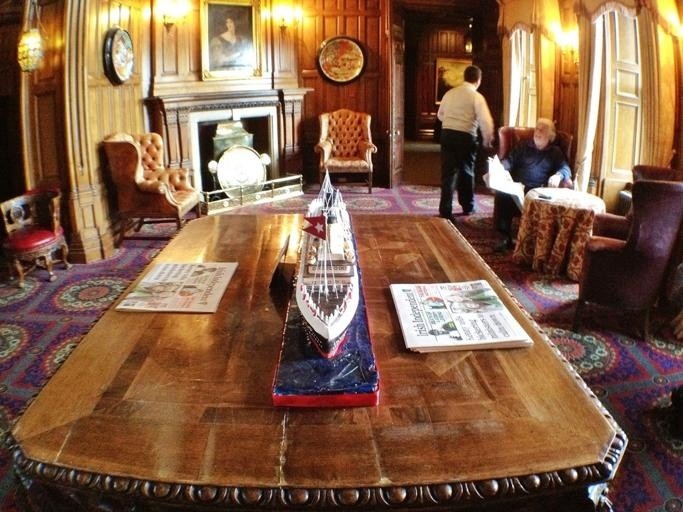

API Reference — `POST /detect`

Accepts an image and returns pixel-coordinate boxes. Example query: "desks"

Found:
[515,186,607,276]
[5,211,630,512]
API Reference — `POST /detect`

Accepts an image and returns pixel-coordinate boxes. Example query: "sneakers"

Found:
[463,206,480,215]
[494,229,516,252]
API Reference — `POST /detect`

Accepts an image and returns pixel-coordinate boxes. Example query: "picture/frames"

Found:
[433,57,473,106]
[199,1,264,82]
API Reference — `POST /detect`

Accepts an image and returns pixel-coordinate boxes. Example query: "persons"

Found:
[436,64,494,220]
[428,323,461,343]
[125,281,180,303]
[210,10,251,70]
[447,287,501,313]
[190,263,216,278]
[436,66,455,103]
[178,287,203,297]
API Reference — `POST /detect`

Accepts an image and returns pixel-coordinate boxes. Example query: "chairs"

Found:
[573,165,683,341]
[312,109,378,195]
[101,132,201,249]
[0,188,74,288]
